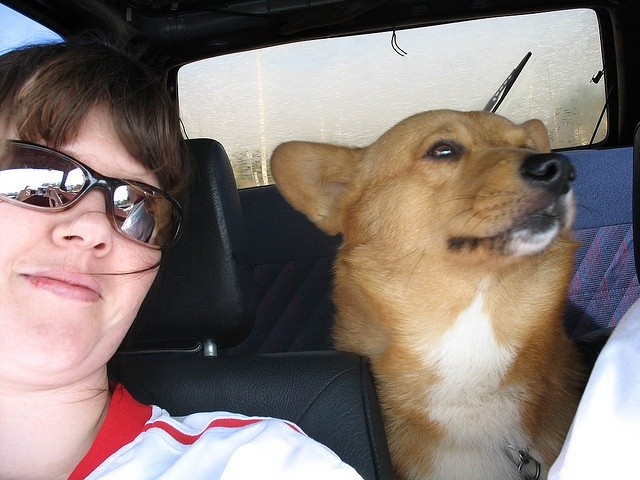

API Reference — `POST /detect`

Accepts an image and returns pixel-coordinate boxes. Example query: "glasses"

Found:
[0,136,183,250]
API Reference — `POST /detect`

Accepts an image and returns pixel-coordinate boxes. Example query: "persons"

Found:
[0,43,364,479]
[548,300,640,479]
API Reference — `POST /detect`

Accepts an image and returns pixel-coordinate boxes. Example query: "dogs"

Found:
[270,109,588,480]
